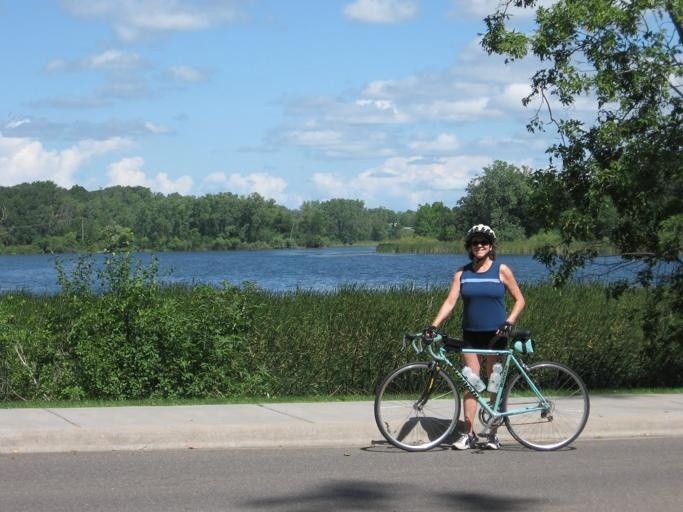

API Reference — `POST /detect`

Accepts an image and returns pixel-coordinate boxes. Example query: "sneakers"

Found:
[484,434,503,450]
[451,431,479,450]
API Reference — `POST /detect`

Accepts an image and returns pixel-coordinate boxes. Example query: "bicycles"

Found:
[374,328,590,452]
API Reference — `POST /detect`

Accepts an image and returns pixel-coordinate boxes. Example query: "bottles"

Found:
[487,364,502,394]
[460,367,486,393]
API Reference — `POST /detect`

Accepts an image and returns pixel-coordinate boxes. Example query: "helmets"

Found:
[467,225,497,243]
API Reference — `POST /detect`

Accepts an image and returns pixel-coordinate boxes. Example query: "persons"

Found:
[420,223,526,451]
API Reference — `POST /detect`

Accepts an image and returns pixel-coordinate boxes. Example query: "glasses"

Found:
[470,237,490,246]
